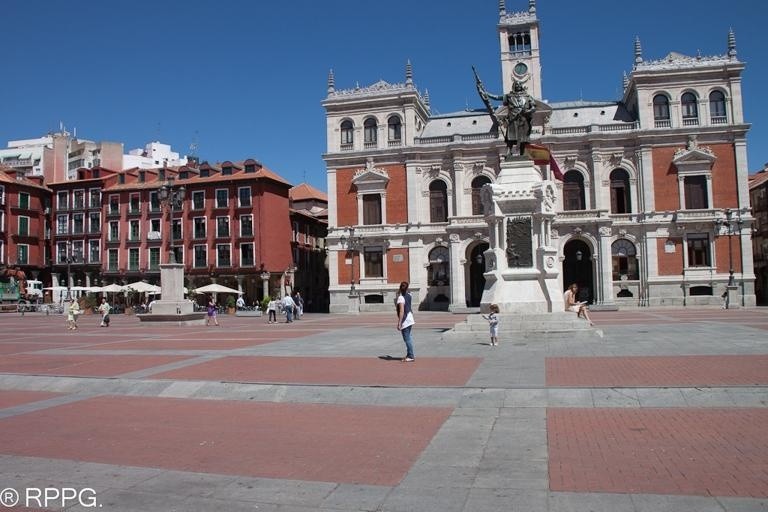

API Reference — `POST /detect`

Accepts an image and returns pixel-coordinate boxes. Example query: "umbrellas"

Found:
[42,281,188,307]
[192,284,245,303]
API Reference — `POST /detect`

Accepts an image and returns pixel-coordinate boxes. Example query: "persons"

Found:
[482,304,500,346]
[69,299,80,330]
[207,296,219,326]
[236,295,246,310]
[253,292,305,324]
[68,310,75,330]
[97,297,111,327]
[487,81,536,159]
[396,282,416,362]
[564,283,594,326]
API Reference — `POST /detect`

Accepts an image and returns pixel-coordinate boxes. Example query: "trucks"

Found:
[0,268,43,313]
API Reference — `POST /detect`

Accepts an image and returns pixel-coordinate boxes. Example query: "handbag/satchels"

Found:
[104,315,109,322]
[73,309,81,315]
[266,308,269,315]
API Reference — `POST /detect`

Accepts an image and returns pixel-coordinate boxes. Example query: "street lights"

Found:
[65,253,78,301]
[340,225,364,298]
[158,173,186,263]
[716,208,744,286]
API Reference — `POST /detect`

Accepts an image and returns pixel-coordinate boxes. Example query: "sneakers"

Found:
[489,342,499,346]
[268,320,293,324]
[68,326,77,330]
[402,357,414,362]
